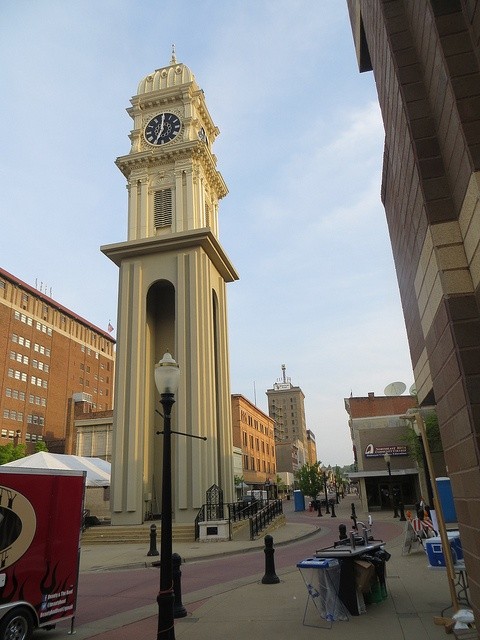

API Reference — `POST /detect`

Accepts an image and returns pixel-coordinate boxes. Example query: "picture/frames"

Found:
[427,558,473,618]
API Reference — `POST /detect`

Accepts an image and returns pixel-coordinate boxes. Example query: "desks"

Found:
[321,464,330,514]
[151,353,179,640]
[399,411,459,632]
[382,451,399,518]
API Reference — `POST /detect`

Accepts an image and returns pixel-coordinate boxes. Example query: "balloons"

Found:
[143,112,183,147]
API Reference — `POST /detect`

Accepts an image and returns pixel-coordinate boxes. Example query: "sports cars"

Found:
[0,465,87,640]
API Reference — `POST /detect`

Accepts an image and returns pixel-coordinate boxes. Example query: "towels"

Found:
[359,540,388,603]
[314,544,367,614]
